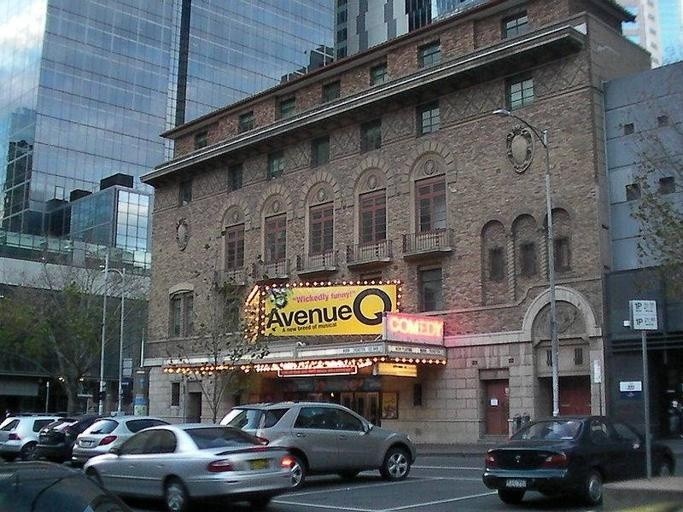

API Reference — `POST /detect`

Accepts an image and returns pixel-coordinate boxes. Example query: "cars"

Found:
[0,461,134,512]
[71,415,176,466]
[82,424,292,512]
[480,415,678,507]
[34,415,107,464]
[0,413,64,462]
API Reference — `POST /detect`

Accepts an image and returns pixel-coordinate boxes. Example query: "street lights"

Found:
[494,109,560,416]
[65,245,125,415]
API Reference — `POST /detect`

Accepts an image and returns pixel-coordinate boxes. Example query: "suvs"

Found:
[217,400,418,482]
